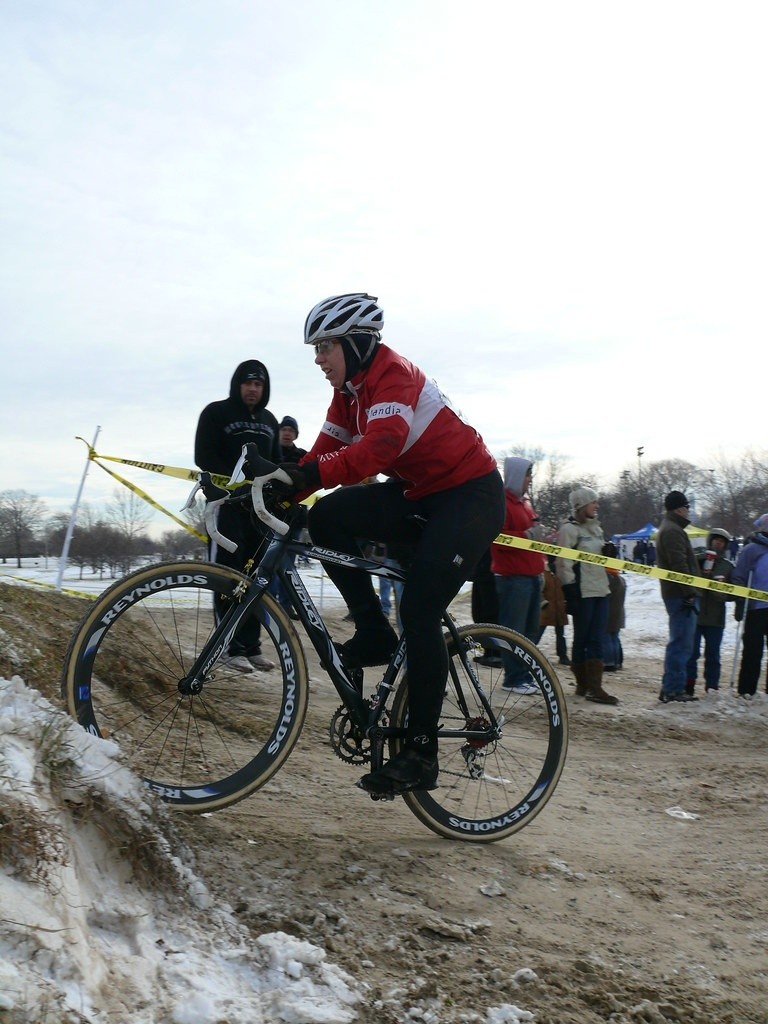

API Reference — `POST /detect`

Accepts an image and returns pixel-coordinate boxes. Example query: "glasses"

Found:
[683,505,689,510]
[280,428,296,432]
[315,339,340,356]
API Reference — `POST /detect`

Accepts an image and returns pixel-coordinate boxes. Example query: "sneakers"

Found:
[359,744,439,794]
[319,635,398,671]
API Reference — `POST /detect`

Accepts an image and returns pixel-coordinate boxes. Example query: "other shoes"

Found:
[216,652,255,674]
[658,689,699,703]
[473,653,504,668]
[575,686,586,696]
[246,655,275,670]
[559,655,571,666]
[501,682,542,695]
[585,689,618,704]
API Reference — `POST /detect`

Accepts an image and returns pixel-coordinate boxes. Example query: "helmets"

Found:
[304,293,383,344]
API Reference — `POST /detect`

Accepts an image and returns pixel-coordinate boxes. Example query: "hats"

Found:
[280,416,299,434]
[569,488,597,510]
[241,365,266,381]
[664,491,689,511]
[706,528,732,550]
[752,514,768,532]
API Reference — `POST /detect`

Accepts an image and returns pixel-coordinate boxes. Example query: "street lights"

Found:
[637,445,646,487]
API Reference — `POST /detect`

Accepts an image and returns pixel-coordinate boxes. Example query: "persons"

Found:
[656,491,768,704]
[195,360,405,672]
[471,457,628,706]
[229,293,506,794]
[633,540,657,574]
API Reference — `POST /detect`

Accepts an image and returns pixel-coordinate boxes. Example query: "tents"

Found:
[612,522,659,565]
[683,524,710,548]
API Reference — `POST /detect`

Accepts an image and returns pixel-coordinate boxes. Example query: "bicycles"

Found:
[60,443,570,842]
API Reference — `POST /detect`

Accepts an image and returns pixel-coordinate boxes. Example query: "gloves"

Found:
[270,460,319,502]
